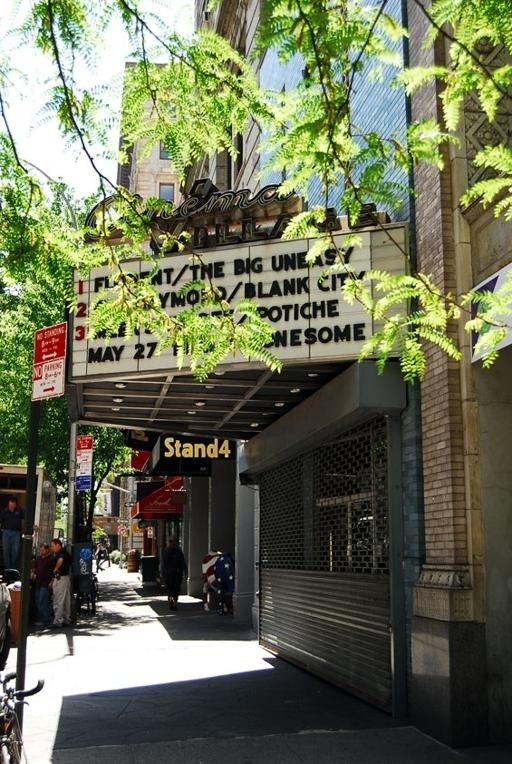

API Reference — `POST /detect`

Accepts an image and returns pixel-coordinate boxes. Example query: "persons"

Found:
[99,538,111,568]
[94,542,106,573]
[0,494,24,570]
[160,535,189,611]
[33,542,54,629]
[49,537,75,626]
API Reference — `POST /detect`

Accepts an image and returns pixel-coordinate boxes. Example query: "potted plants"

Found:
[127,549,141,572]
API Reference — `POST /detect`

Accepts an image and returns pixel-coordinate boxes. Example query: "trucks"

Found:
[1,461,65,568]
[345,513,389,574]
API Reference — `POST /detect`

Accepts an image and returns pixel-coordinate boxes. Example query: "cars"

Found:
[0,567,21,669]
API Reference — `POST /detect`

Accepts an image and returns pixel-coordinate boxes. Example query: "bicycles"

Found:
[1,669,49,764]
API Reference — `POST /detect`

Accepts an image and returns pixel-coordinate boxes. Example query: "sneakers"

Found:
[167,603,176,611]
[53,617,72,624]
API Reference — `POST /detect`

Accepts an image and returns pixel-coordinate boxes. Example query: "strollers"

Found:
[202,552,233,616]
